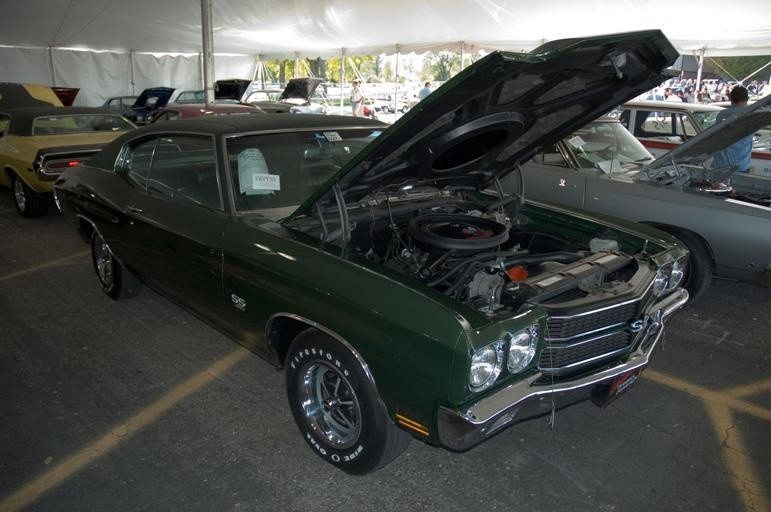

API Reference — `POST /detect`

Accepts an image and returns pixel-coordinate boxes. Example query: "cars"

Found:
[606,97,771,179]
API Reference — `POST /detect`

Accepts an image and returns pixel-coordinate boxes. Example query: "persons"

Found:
[709,85,753,175]
[349,79,361,116]
[357,79,364,117]
[649,78,771,103]
[418,80,434,101]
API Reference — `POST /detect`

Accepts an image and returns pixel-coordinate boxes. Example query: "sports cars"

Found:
[0,83,140,220]
[84,77,416,175]
[482,94,771,307]
[51,27,695,474]
